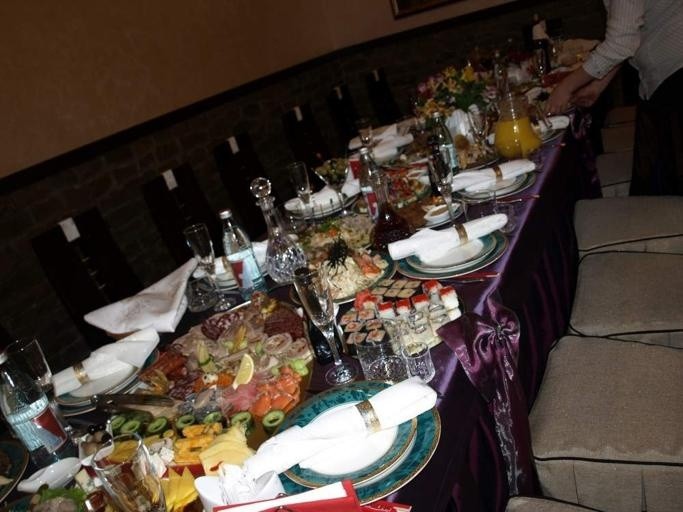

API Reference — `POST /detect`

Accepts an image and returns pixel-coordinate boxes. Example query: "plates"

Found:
[360,176,436,187]
[54,348,159,418]
[451,171,536,205]
[273,381,441,506]
[192,252,269,290]
[17,457,82,493]
[538,116,570,131]
[291,251,398,305]
[396,230,508,280]
[284,194,358,220]
[409,143,498,171]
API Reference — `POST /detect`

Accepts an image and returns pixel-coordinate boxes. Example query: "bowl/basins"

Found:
[424,202,460,223]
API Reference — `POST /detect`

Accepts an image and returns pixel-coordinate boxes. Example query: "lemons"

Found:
[232,353,255,391]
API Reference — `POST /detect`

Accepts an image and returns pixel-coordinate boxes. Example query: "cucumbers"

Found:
[108,409,286,439]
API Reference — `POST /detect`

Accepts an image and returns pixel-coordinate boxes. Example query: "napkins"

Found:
[282,179,360,212]
[82,257,201,334]
[48,327,162,396]
[437,158,537,193]
[385,211,508,260]
[347,124,414,161]
[243,375,437,482]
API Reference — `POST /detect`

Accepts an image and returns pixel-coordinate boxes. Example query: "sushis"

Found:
[339,278,460,356]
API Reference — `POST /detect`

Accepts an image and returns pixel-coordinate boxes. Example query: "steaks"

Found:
[202,301,305,343]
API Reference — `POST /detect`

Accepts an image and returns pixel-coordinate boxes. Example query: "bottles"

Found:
[359,148,386,223]
[1,352,77,462]
[366,170,411,258]
[220,209,267,301]
[431,112,460,176]
[250,177,307,286]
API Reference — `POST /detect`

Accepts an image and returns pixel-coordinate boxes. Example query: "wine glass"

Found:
[288,160,316,227]
[429,148,462,228]
[294,267,348,365]
[186,222,237,311]
[313,152,357,219]
[292,259,358,385]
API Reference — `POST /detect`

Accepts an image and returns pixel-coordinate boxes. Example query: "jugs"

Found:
[494,91,554,159]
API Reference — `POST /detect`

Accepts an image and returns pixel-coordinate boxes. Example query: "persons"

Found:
[542,0,682,197]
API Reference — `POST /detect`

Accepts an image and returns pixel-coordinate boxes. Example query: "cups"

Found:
[187,275,220,311]
[354,318,408,385]
[401,342,436,384]
[4,336,60,416]
[80,445,112,479]
[90,435,168,512]
[355,118,377,160]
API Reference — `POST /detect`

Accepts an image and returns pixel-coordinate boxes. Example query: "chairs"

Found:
[572,196,682,257]
[277,69,401,185]
[568,250,681,351]
[32,205,146,354]
[527,334,682,511]
[598,103,635,198]
[145,124,281,263]
